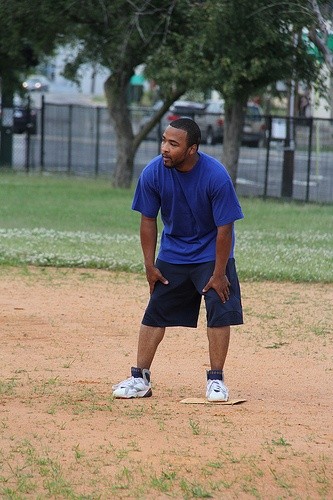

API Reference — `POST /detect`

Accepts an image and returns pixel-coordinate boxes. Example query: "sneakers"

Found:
[206,369,228,401]
[112,367,152,398]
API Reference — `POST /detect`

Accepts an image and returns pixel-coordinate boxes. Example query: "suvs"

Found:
[137,100,208,144]
[204,99,266,148]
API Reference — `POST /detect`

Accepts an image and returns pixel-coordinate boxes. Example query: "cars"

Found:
[22,74,49,93]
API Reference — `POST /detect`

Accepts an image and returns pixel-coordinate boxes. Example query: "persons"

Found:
[111,116,245,405]
